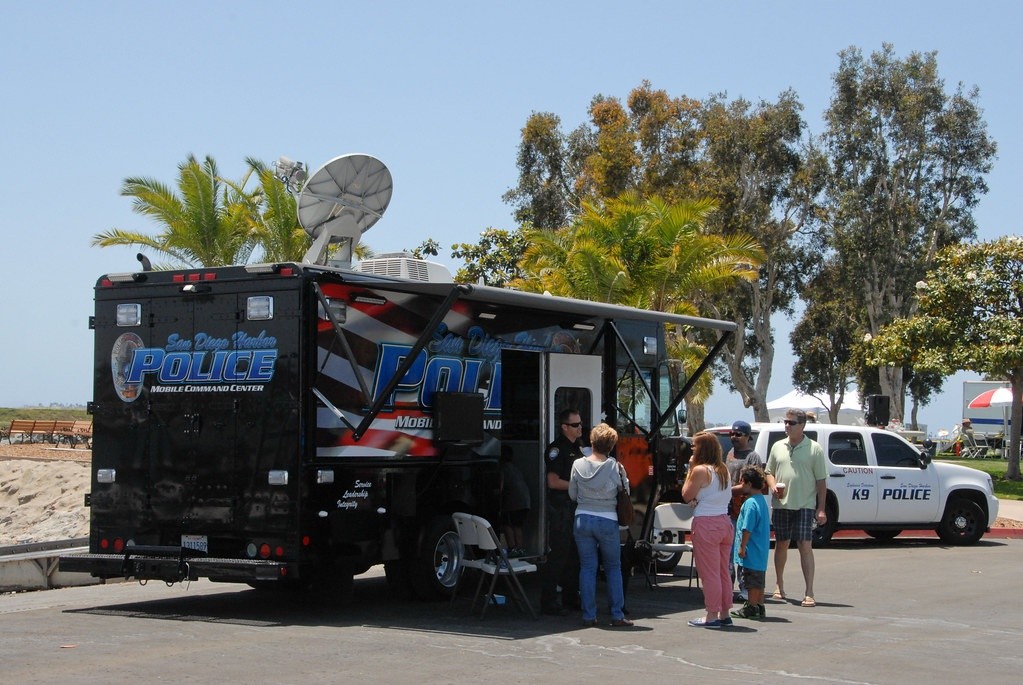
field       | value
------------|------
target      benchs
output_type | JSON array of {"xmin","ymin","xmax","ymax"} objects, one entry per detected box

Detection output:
[{"xmin": 0, "ymin": 419, "xmax": 93, "ymax": 449}]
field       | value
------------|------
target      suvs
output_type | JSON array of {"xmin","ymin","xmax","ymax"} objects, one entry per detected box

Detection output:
[{"xmin": 691, "ymin": 422, "xmax": 998, "ymax": 545}]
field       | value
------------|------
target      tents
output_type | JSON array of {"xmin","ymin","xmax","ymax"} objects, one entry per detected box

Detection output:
[{"xmin": 763, "ymin": 390, "xmax": 868, "ymax": 421}]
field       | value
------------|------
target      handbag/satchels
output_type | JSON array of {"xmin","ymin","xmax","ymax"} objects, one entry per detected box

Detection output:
[{"xmin": 616, "ymin": 473, "xmax": 634, "ymax": 527}]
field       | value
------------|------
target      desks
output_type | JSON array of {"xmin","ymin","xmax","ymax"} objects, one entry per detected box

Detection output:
[{"xmin": 987, "ymin": 437, "xmax": 1004, "ymax": 459}]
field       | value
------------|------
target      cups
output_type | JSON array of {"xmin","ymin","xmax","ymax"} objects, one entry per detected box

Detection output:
[{"xmin": 777, "ymin": 483, "xmax": 785, "ymax": 499}]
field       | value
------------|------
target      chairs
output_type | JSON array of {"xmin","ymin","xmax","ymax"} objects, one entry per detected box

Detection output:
[
  {"xmin": 597, "ymin": 503, "xmax": 699, "ymax": 591},
  {"xmin": 451, "ymin": 513, "xmax": 539, "ymax": 622},
  {"xmin": 961, "ymin": 433, "xmax": 1023, "ymax": 459}
]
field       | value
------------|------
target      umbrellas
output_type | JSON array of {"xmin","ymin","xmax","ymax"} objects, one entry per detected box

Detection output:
[{"xmin": 968, "ymin": 387, "xmax": 1023, "ymax": 435}]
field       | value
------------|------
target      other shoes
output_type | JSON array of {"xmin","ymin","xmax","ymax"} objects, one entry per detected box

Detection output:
[
  {"xmin": 772, "ymin": 590, "xmax": 785, "ymax": 599},
  {"xmin": 612, "ymin": 619, "xmax": 634, "ymax": 626},
  {"xmin": 562, "ymin": 599, "xmax": 581, "ymax": 612},
  {"xmin": 584, "ymin": 619, "xmax": 604, "ymax": 627},
  {"xmin": 543, "ymin": 603, "xmax": 566, "ymax": 616},
  {"xmin": 801, "ymin": 595, "xmax": 816, "ymax": 606}
]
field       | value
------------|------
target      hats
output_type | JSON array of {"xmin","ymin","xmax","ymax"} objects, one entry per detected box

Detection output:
[
  {"xmin": 961, "ymin": 419, "xmax": 971, "ymax": 423},
  {"xmin": 732, "ymin": 420, "xmax": 754, "ymax": 441},
  {"xmin": 806, "ymin": 411, "xmax": 816, "ymax": 418}
]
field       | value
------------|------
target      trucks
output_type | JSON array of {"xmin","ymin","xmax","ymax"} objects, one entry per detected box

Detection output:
[{"xmin": 84, "ymin": 253, "xmax": 738, "ymax": 604}]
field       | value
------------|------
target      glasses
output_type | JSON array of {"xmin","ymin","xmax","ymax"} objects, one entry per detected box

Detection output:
[
  {"xmin": 691, "ymin": 443, "xmax": 696, "ymax": 447},
  {"xmin": 565, "ymin": 421, "xmax": 582, "ymax": 428},
  {"xmin": 784, "ymin": 419, "xmax": 800, "ymax": 425},
  {"xmin": 729, "ymin": 431, "xmax": 746, "ymax": 437}
]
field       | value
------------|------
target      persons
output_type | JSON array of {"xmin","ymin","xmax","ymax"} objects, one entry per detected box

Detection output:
[
  {"xmin": 725, "ymin": 421, "xmax": 763, "ymax": 602},
  {"xmin": 543, "ymin": 410, "xmax": 582, "ymax": 616},
  {"xmin": 766, "ymin": 408, "xmax": 828, "ymax": 607},
  {"xmin": 731, "ymin": 465, "xmax": 770, "ymax": 620},
  {"xmin": 681, "ymin": 431, "xmax": 733, "ymax": 628},
  {"xmin": 569, "ymin": 423, "xmax": 633, "ymax": 626},
  {"xmin": 961, "ymin": 418, "xmax": 989, "ymax": 458},
  {"xmin": 499, "ymin": 446, "xmax": 531, "ymax": 560}
]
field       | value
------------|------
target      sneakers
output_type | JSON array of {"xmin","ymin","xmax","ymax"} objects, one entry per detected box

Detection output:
[
  {"xmin": 718, "ymin": 617, "xmax": 733, "ymax": 626},
  {"xmin": 688, "ymin": 617, "xmax": 720, "ymax": 628},
  {"xmin": 730, "ymin": 601, "xmax": 765, "ymax": 619}
]
